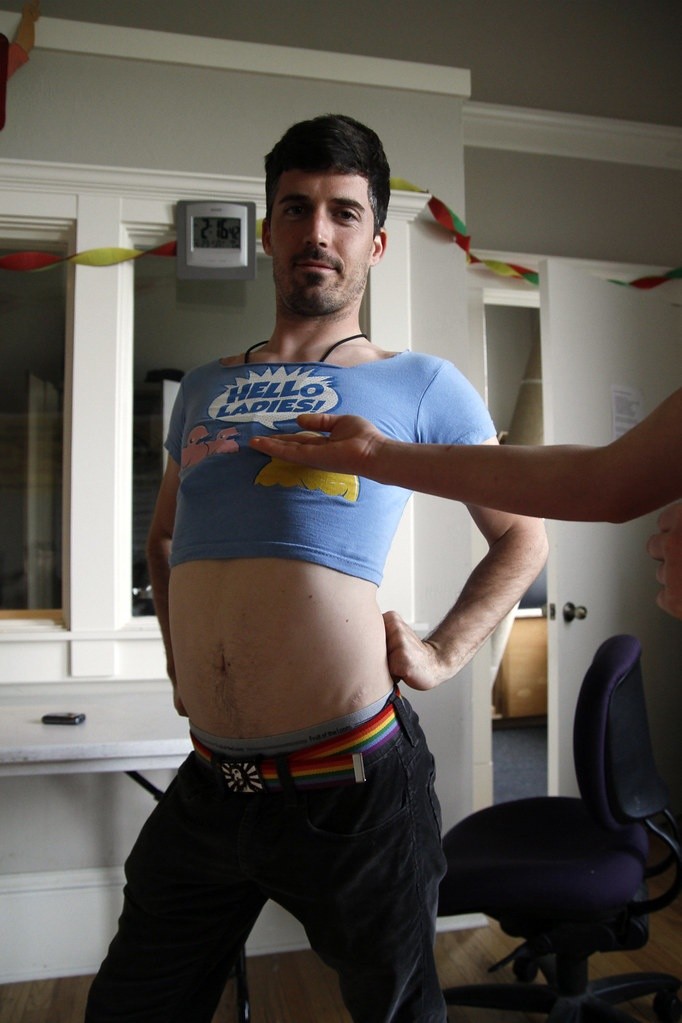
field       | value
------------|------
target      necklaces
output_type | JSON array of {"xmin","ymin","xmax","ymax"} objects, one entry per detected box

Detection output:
[{"xmin": 244, "ymin": 333, "xmax": 365, "ymax": 364}]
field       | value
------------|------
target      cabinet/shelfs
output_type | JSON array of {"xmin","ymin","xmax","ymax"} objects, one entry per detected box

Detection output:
[{"xmin": 133, "ymin": 379, "xmax": 181, "ymax": 481}]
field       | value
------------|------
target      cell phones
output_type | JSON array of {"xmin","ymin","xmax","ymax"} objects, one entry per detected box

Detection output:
[{"xmin": 41, "ymin": 712, "xmax": 85, "ymax": 724}]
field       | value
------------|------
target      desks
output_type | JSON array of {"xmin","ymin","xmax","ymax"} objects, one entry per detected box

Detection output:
[{"xmin": 0, "ymin": 684, "xmax": 249, "ymax": 1023}]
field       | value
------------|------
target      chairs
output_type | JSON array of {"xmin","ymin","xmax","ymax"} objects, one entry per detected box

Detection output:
[{"xmin": 438, "ymin": 635, "xmax": 682, "ymax": 1023}]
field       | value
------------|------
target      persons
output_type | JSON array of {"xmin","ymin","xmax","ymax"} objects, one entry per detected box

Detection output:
[
  {"xmin": 78, "ymin": 114, "xmax": 547, "ymax": 1023},
  {"xmin": 249, "ymin": 388, "xmax": 682, "ymax": 621}
]
